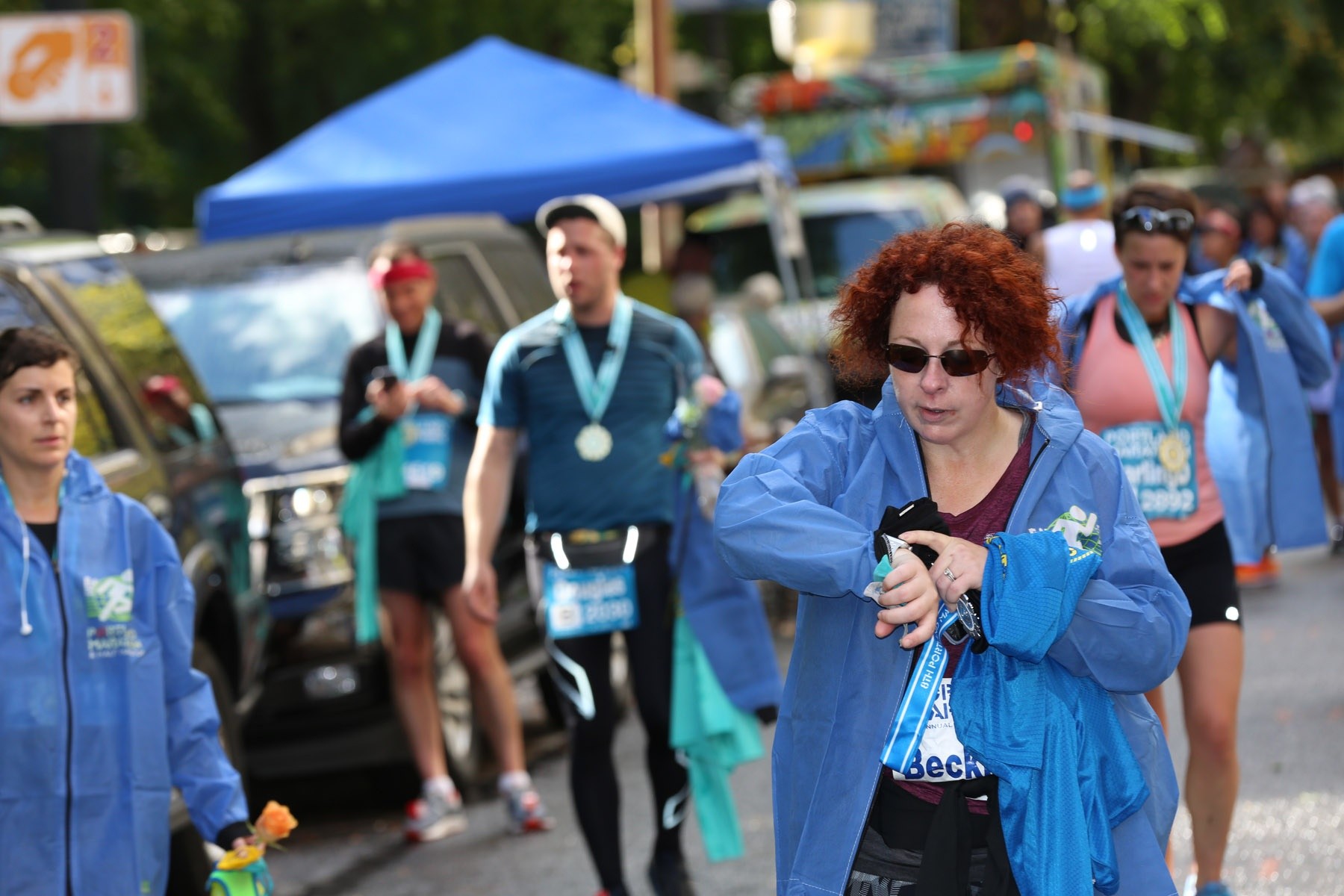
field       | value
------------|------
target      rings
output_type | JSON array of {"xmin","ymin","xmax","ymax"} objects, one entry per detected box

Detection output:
[{"xmin": 944, "ymin": 568, "xmax": 955, "ymax": 582}]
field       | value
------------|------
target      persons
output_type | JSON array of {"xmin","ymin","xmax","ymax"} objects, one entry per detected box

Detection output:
[
  {"xmin": 1054, "ymin": 182, "xmax": 1333, "ymax": 896},
  {"xmin": 998, "ymin": 169, "xmax": 1344, "ymax": 586},
  {"xmin": 336, "ymin": 242, "xmax": 553, "ymax": 842},
  {"xmin": 710, "ymin": 221, "xmax": 1193, "ymax": 896},
  {"xmin": 0, "ymin": 327, "xmax": 259, "ymax": 896},
  {"xmin": 462, "ymin": 194, "xmax": 725, "ymax": 895},
  {"xmin": 141, "ymin": 372, "xmax": 258, "ymax": 721}
]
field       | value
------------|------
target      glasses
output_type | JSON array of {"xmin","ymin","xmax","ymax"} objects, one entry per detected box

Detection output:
[
  {"xmin": 1122, "ymin": 205, "xmax": 1194, "ymax": 234},
  {"xmin": 884, "ymin": 341, "xmax": 998, "ymax": 378}
]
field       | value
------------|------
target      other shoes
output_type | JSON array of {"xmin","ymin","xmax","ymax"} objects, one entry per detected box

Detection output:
[
  {"xmin": 501, "ymin": 783, "xmax": 553, "ymax": 831},
  {"xmin": 1233, "ymin": 555, "xmax": 1279, "ymax": 588},
  {"xmin": 405, "ymin": 789, "xmax": 466, "ymax": 846},
  {"xmin": 1184, "ymin": 873, "xmax": 1232, "ymax": 896}
]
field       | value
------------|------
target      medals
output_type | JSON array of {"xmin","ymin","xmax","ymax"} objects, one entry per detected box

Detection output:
[
  {"xmin": 1159, "ymin": 428, "xmax": 1188, "ymax": 472},
  {"xmin": 405, "ymin": 423, "xmax": 418, "ymax": 445},
  {"xmin": 574, "ymin": 423, "xmax": 612, "ymax": 462}
]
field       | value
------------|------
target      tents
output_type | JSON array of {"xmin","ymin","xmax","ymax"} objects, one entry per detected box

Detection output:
[{"xmin": 191, "ymin": 35, "xmax": 831, "ymax": 411}]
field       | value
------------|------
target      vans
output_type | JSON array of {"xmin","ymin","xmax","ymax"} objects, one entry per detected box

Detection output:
[{"xmin": 0, "ymin": 36, "xmax": 1227, "ymax": 896}]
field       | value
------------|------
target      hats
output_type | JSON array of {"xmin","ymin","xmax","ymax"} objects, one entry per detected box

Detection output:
[{"xmin": 534, "ymin": 193, "xmax": 629, "ymax": 251}]
